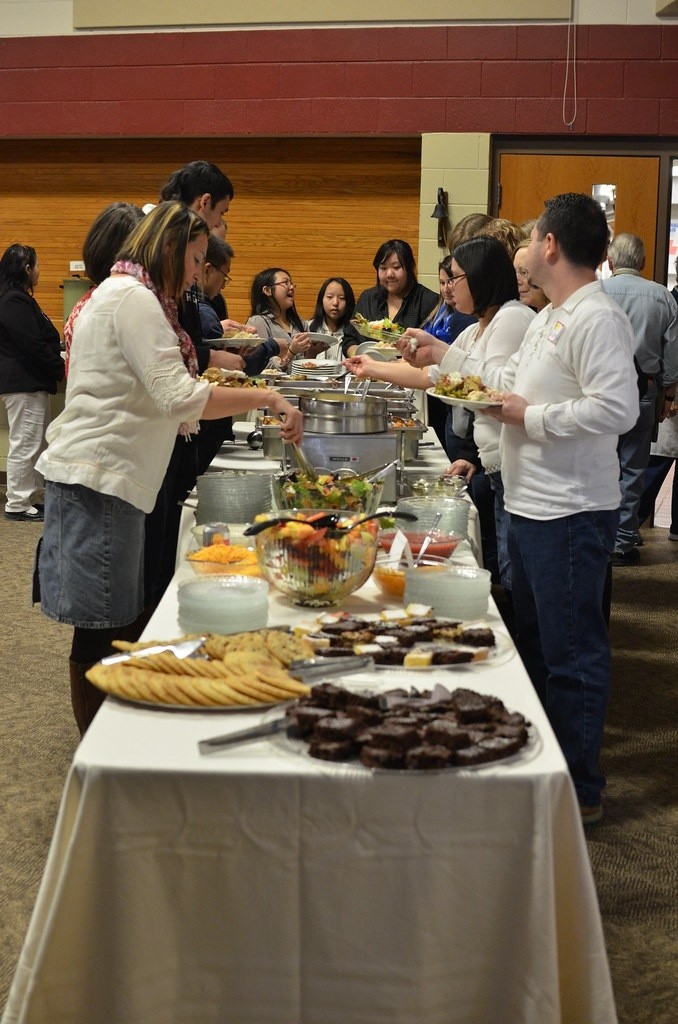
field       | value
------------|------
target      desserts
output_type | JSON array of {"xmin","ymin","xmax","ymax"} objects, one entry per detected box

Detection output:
[
  {"xmin": 292, "ymin": 603, "xmax": 496, "ymax": 667},
  {"xmin": 285, "ymin": 681, "xmax": 526, "ymax": 770}
]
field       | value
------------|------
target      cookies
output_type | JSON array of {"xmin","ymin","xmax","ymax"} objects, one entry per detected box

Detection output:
[{"xmin": 85, "ymin": 628, "xmax": 316, "ymax": 708}]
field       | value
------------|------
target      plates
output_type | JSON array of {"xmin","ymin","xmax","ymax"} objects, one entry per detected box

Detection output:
[
  {"xmin": 402, "ymin": 564, "xmax": 492, "ymax": 621},
  {"xmin": 258, "ymin": 372, "xmax": 287, "ymax": 380},
  {"xmin": 394, "ymin": 495, "xmax": 472, "ymax": 554},
  {"xmin": 350, "ymin": 320, "xmax": 411, "ymax": 344},
  {"xmin": 204, "ymin": 337, "xmax": 266, "ymax": 349},
  {"xmin": 366, "ymin": 616, "xmax": 515, "ymax": 674},
  {"xmin": 425, "ymin": 386, "xmax": 504, "ymax": 409},
  {"xmin": 355, "ymin": 341, "xmax": 397, "ymax": 362},
  {"xmin": 291, "ymin": 332, "xmax": 339, "ymax": 347},
  {"xmin": 195, "ymin": 473, "xmax": 279, "ymax": 525},
  {"xmin": 261, "ymin": 689, "xmax": 540, "ymax": 776},
  {"xmin": 176, "ymin": 573, "xmax": 270, "ymax": 639},
  {"xmin": 290, "ymin": 358, "xmax": 342, "ymax": 376}
]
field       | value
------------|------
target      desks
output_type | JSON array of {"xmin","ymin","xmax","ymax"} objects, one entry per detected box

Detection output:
[{"xmin": 1, "ymin": 419, "xmax": 621, "ymax": 1024}]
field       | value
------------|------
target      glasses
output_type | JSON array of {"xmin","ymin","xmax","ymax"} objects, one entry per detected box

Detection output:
[
  {"xmin": 516, "ymin": 266, "xmax": 529, "ymax": 279},
  {"xmin": 211, "ymin": 263, "xmax": 232, "ymax": 285},
  {"xmin": 447, "ymin": 274, "xmax": 467, "ymax": 287},
  {"xmin": 261, "ymin": 280, "xmax": 296, "ymax": 294}
]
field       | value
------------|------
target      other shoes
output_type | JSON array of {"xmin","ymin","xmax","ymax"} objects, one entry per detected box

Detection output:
[
  {"xmin": 611, "ymin": 547, "xmax": 641, "ymax": 567},
  {"xmin": 668, "ymin": 533, "xmax": 678, "ymax": 540},
  {"xmin": 6, "ymin": 504, "xmax": 45, "ymax": 522}
]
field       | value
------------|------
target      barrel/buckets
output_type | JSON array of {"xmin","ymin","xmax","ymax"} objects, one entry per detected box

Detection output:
[{"xmin": 298, "ymin": 393, "xmax": 388, "ymax": 434}]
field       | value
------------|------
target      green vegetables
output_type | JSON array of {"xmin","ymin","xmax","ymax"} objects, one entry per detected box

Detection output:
[
  {"xmin": 433, "ymin": 372, "xmax": 477, "ymax": 401},
  {"xmin": 351, "ymin": 312, "xmax": 404, "ymax": 341},
  {"xmin": 275, "ymin": 471, "xmax": 396, "ymax": 528}
]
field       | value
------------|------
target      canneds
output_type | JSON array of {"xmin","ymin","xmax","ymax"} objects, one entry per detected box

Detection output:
[{"xmin": 202, "ymin": 521, "xmax": 230, "ymax": 548}]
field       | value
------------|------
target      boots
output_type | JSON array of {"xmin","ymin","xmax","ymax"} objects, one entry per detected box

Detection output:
[{"xmin": 68, "ymin": 656, "xmax": 107, "ymax": 742}]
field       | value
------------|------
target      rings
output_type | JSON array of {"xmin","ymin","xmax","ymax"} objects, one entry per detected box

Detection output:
[{"xmin": 446, "ymin": 468, "xmax": 449, "ymax": 471}]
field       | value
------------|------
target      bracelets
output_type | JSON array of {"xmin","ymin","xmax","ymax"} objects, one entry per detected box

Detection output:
[
  {"xmin": 289, "ymin": 349, "xmax": 296, "ymax": 355},
  {"xmin": 664, "ymin": 395, "xmax": 675, "ymax": 402}
]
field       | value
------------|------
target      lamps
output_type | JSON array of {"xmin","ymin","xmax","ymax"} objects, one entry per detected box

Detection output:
[{"xmin": 431, "ymin": 187, "xmax": 451, "ymax": 248}]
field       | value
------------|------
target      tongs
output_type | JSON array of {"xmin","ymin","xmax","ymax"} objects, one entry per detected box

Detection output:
[
  {"xmin": 278, "ymin": 412, "xmax": 319, "ymax": 483},
  {"xmin": 287, "ymin": 651, "xmax": 375, "ymax": 684},
  {"xmin": 341, "ymin": 458, "xmax": 402, "ymax": 485}
]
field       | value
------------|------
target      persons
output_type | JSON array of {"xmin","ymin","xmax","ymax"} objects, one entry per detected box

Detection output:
[
  {"xmin": 341, "ymin": 239, "xmax": 440, "ymax": 359},
  {"xmin": 419, "ymin": 213, "xmax": 551, "ymax": 583},
  {"xmin": 143, "ymin": 160, "xmax": 289, "ymax": 611},
  {"xmin": 0, "ymin": 243, "xmax": 64, "ymax": 522},
  {"xmin": 340, "ymin": 235, "xmax": 537, "ymax": 642},
  {"xmin": 395, "ymin": 192, "xmax": 640, "ymax": 824},
  {"xmin": 245, "ymin": 268, "xmax": 329, "ymax": 375},
  {"xmin": 33, "ymin": 200, "xmax": 303, "ymax": 741},
  {"xmin": 307, "ymin": 277, "xmax": 355, "ymax": 362},
  {"xmin": 63, "ymin": 203, "xmax": 147, "ymax": 379},
  {"xmin": 601, "ymin": 233, "xmax": 678, "ymax": 565}
]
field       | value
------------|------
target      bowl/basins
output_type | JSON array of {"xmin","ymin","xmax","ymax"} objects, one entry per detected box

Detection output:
[
  {"xmin": 183, "ymin": 548, "xmax": 263, "ymax": 578},
  {"xmin": 254, "ymin": 509, "xmax": 380, "ymax": 609},
  {"xmin": 376, "ymin": 526, "xmax": 465, "ymax": 567},
  {"xmin": 405, "ymin": 472, "xmax": 469, "ymax": 499},
  {"xmin": 371, "ymin": 552, "xmax": 453, "ymax": 603}
]
